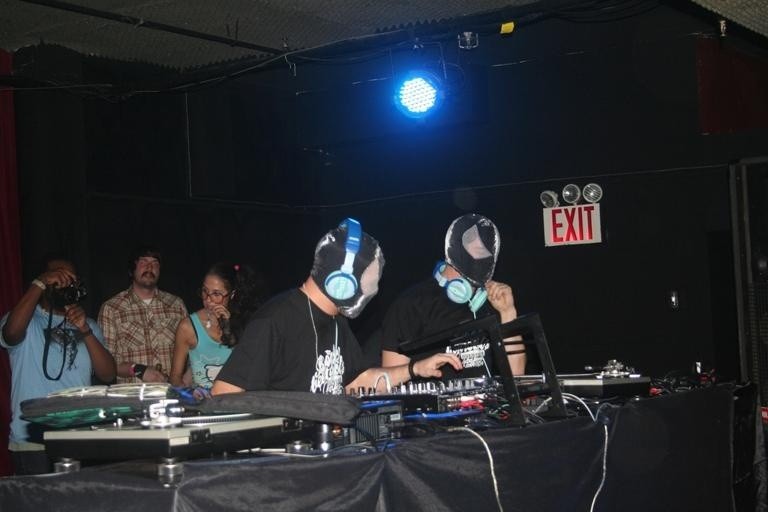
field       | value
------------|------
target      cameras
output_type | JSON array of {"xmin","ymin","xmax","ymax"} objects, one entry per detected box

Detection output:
[{"xmin": 44, "ymin": 279, "xmax": 87, "ymax": 307}]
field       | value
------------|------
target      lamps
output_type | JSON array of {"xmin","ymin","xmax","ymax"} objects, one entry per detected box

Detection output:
[
  {"xmin": 539, "ymin": 181, "xmax": 605, "ymax": 209},
  {"xmin": 387, "ymin": 40, "xmax": 452, "ymax": 122}
]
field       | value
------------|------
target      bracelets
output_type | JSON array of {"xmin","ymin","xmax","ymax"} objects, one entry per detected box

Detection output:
[
  {"xmin": 31, "ymin": 279, "xmax": 48, "ymax": 291},
  {"xmin": 409, "ymin": 358, "xmax": 420, "ymax": 380},
  {"xmin": 129, "ymin": 362, "xmax": 145, "ymax": 381},
  {"xmin": 82, "ymin": 329, "xmax": 93, "ymax": 337}
]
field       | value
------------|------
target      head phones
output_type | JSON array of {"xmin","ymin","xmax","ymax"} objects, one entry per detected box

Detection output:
[
  {"xmin": 324, "ymin": 217, "xmax": 363, "ymax": 300},
  {"xmin": 433, "ymin": 260, "xmax": 489, "ymax": 313}
]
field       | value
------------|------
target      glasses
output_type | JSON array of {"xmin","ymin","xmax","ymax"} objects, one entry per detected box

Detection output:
[{"xmin": 198, "ymin": 287, "xmax": 231, "ymax": 305}]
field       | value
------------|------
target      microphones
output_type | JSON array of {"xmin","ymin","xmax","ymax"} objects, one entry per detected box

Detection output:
[{"xmin": 465, "ymin": 296, "xmax": 476, "ymax": 319}]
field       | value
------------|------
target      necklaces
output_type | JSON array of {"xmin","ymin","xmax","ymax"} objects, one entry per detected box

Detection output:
[{"xmin": 206, "ymin": 312, "xmax": 212, "ymax": 329}]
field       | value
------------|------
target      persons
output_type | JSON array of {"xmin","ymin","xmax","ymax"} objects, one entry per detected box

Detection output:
[
  {"xmin": 381, "ymin": 215, "xmax": 528, "ymax": 378},
  {"xmin": 169, "ymin": 261, "xmax": 260, "ymax": 394},
  {"xmin": 210, "ymin": 218, "xmax": 463, "ymax": 404},
  {"xmin": 97, "ymin": 240, "xmax": 189, "ymax": 386},
  {"xmin": 0, "ymin": 250, "xmax": 117, "ymax": 474}
]
